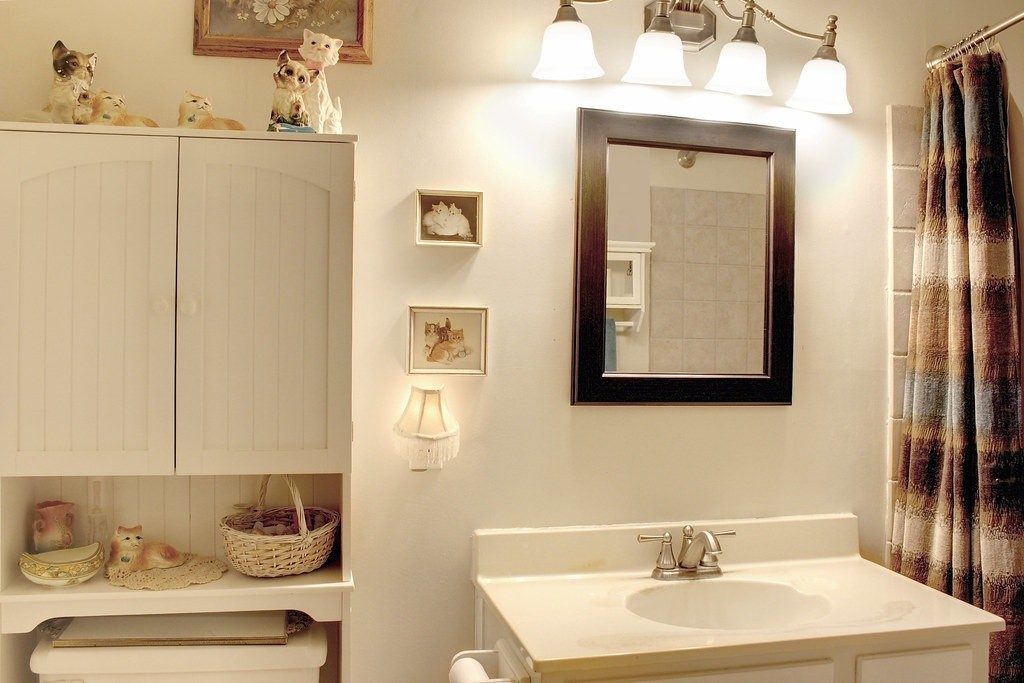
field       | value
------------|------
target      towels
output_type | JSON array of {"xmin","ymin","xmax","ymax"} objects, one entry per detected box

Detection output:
[{"xmin": 605, "ymin": 317, "xmax": 616, "ymax": 370}]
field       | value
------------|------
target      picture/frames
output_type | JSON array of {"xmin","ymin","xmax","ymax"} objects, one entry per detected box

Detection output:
[
  {"xmin": 568, "ymin": 107, "xmax": 799, "ymax": 406},
  {"xmin": 413, "ymin": 187, "xmax": 484, "ymax": 246},
  {"xmin": 193, "ymin": 0, "xmax": 376, "ymax": 67},
  {"xmin": 406, "ymin": 303, "xmax": 490, "ymax": 376}
]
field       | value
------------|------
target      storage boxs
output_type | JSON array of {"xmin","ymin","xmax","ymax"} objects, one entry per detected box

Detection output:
[{"xmin": 29, "ymin": 622, "xmax": 327, "ymax": 683}]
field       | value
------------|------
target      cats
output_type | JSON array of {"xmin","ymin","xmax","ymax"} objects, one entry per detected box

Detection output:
[
  {"xmin": 175, "ymin": 90, "xmax": 244, "ymax": 131},
  {"xmin": 266, "ymin": 28, "xmax": 344, "ymax": 134},
  {"xmin": 103, "ymin": 524, "xmax": 187, "ymax": 578},
  {"xmin": 16, "ymin": 40, "xmax": 161, "ymax": 127}
]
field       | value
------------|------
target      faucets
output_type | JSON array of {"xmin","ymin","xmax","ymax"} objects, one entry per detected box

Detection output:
[{"xmin": 678, "ymin": 525, "xmax": 722, "ymax": 579}]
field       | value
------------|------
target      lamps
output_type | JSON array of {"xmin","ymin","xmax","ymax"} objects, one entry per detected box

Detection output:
[
  {"xmin": 393, "ymin": 385, "xmax": 463, "ymax": 473},
  {"xmin": 530, "ymin": 0, "xmax": 607, "ymax": 82},
  {"xmin": 786, "ymin": 14, "xmax": 855, "ymax": 115},
  {"xmin": 702, "ymin": 0, "xmax": 773, "ymax": 98},
  {"xmin": 623, "ymin": 0, "xmax": 693, "ymax": 87}
]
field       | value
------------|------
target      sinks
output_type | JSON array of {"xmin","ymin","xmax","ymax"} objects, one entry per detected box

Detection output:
[{"xmin": 624, "ymin": 579, "xmax": 812, "ymax": 632}]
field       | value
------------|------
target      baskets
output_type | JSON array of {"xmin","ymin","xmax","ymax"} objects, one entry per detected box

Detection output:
[{"xmin": 219, "ymin": 474, "xmax": 341, "ymax": 577}]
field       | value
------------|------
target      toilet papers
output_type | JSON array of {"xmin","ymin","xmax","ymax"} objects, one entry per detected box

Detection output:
[{"xmin": 447, "ymin": 655, "xmax": 491, "ymax": 683}]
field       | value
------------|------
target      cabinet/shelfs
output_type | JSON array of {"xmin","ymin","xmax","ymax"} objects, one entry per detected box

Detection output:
[
  {"xmin": 605, "ymin": 236, "xmax": 657, "ymax": 331},
  {"xmin": 0, "ymin": 122, "xmax": 358, "ymax": 683},
  {"xmin": 478, "ymin": 601, "xmax": 989, "ymax": 681}
]
